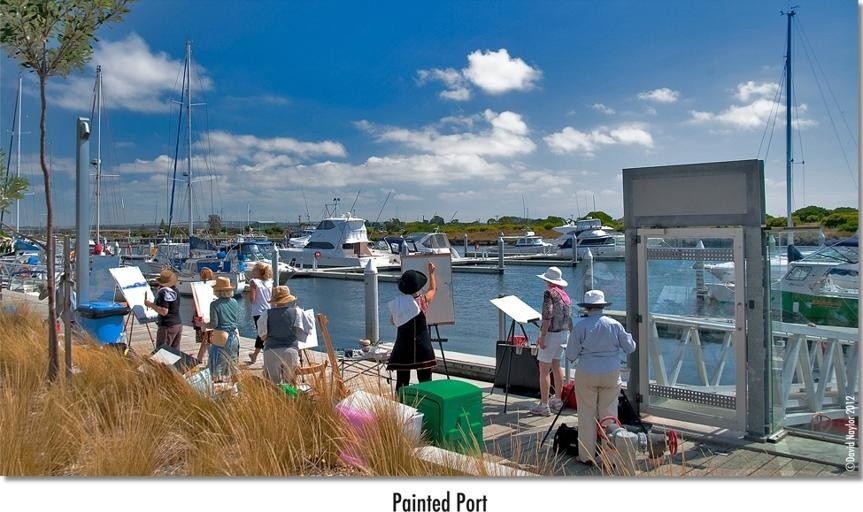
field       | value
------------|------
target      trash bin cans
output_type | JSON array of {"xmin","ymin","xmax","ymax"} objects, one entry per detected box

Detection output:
[{"xmin": 74, "ymin": 301, "xmax": 130, "ymax": 346}]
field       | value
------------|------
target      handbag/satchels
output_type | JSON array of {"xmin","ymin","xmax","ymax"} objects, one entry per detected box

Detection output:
[
  {"xmin": 553, "ymin": 423, "xmax": 577, "ymax": 458},
  {"xmin": 560, "ymin": 381, "xmax": 576, "ymax": 409}
]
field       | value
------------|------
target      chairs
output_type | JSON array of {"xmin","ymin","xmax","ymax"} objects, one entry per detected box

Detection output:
[{"xmin": 290, "ymin": 360, "xmax": 328, "ymax": 388}]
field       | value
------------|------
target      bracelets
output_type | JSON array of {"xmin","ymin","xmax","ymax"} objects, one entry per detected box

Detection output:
[{"xmin": 541, "ymin": 335, "xmax": 546, "ymax": 336}]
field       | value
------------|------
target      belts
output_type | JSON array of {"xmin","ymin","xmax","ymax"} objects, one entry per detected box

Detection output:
[{"xmin": 547, "ymin": 329, "xmax": 564, "ymax": 333}]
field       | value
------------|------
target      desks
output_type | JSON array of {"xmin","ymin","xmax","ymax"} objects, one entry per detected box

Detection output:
[{"xmin": 338, "ymin": 350, "xmax": 393, "ymax": 400}]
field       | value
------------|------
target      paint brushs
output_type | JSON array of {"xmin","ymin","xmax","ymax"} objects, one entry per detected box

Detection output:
[{"xmin": 146, "ymin": 307, "xmax": 149, "ymax": 311}]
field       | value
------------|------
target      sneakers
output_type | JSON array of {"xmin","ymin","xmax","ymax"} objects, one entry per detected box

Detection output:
[
  {"xmin": 547, "ymin": 399, "xmax": 562, "ymax": 412},
  {"xmin": 575, "ymin": 456, "xmax": 593, "ymax": 466},
  {"xmin": 248, "ymin": 352, "xmax": 256, "ymax": 364},
  {"xmin": 529, "ymin": 403, "xmax": 550, "ymax": 418}
]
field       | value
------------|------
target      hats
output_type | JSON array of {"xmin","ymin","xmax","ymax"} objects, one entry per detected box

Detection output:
[
  {"xmin": 211, "ymin": 277, "xmax": 236, "ymax": 291},
  {"xmin": 94, "ymin": 244, "xmax": 102, "ymax": 253},
  {"xmin": 156, "ymin": 270, "xmax": 177, "ymax": 287},
  {"xmin": 267, "ymin": 286, "xmax": 297, "ymax": 305},
  {"xmin": 575, "ymin": 290, "xmax": 612, "ymax": 309},
  {"xmin": 534, "ymin": 266, "xmax": 568, "ymax": 287},
  {"xmin": 397, "ymin": 270, "xmax": 427, "ymax": 294}
]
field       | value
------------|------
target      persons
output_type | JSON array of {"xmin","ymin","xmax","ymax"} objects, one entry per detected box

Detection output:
[
  {"xmin": 144, "ymin": 270, "xmax": 183, "ymax": 351},
  {"xmin": 257, "ymin": 285, "xmax": 300, "ymax": 385},
  {"xmin": 193, "ymin": 267, "xmax": 214, "ymax": 361},
  {"xmin": 386, "ymin": 261, "xmax": 438, "ymax": 392},
  {"xmin": 248, "ymin": 262, "xmax": 274, "ymax": 363},
  {"xmin": 529, "ymin": 266, "xmax": 573, "ymax": 417},
  {"xmin": 195, "ymin": 275, "xmax": 240, "ymax": 382},
  {"xmin": 565, "ymin": 289, "xmax": 637, "ymax": 467}
]
